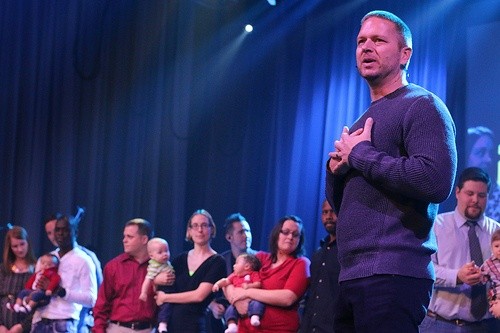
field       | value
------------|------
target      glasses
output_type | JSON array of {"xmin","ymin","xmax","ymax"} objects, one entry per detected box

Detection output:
[
  {"xmin": 279, "ymin": 229, "xmax": 301, "ymax": 238},
  {"xmin": 189, "ymin": 222, "xmax": 213, "ymax": 230}
]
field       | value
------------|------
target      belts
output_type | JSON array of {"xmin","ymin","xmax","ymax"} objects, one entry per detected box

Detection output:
[
  {"xmin": 427, "ymin": 309, "xmax": 491, "ymax": 327},
  {"xmin": 110, "ymin": 320, "xmax": 155, "ymax": 331},
  {"xmin": 37, "ymin": 317, "xmax": 76, "ymax": 326}
]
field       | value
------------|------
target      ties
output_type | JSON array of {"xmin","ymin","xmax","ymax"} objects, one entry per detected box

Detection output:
[{"xmin": 465, "ymin": 220, "xmax": 488, "ymax": 320}]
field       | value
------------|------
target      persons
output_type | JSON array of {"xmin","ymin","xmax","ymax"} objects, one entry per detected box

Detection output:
[
  {"xmin": 207, "ymin": 212, "xmax": 258, "ymax": 319},
  {"xmin": 488, "ymin": 304, "xmax": 500, "ymax": 320},
  {"xmin": 301, "ymin": 197, "xmax": 353, "ymax": 333},
  {"xmin": 224, "ymin": 215, "xmax": 312, "ymax": 333},
  {"xmin": 31, "ymin": 217, "xmax": 103, "ymax": 333},
  {"xmin": 92, "ymin": 219, "xmax": 157, "ymax": 333},
  {"xmin": 0, "ymin": 225, "xmax": 37, "ymax": 333},
  {"xmin": 14, "ymin": 253, "xmax": 59, "ymax": 314},
  {"xmin": 210, "ymin": 254, "xmax": 266, "ymax": 333},
  {"xmin": 34, "ymin": 219, "xmax": 104, "ymax": 297},
  {"xmin": 419, "ymin": 167, "xmax": 499, "ymax": 333},
  {"xmin": 325, "ymin": 10, "xmax": 457, "ymax": 333},
  {"xmin": 153, "ymin": 209, "xmax": 227, "ymax": 333},
  {"xmin": 141, "ymin": 237, "xmax": 175, "ymax": 333},
  {"xmin": 471, "ymin": 229, "xmax": 499, "ymax": 320}
]
